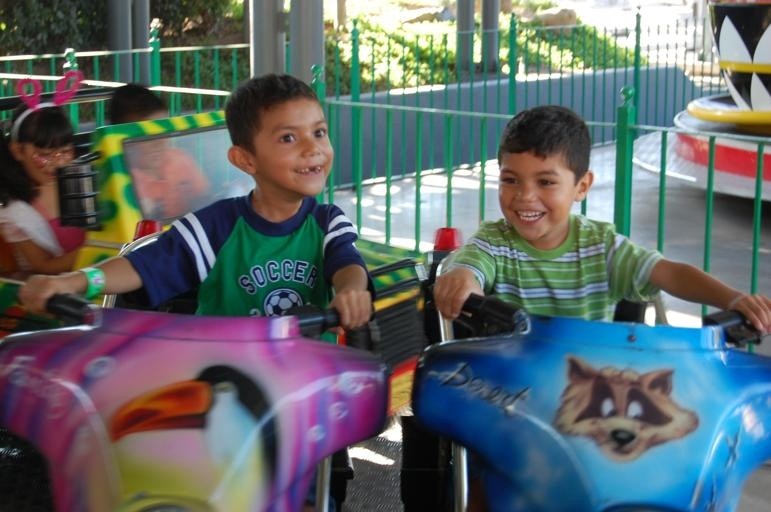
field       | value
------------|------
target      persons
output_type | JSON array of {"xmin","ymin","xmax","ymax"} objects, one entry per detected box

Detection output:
[
  {"xmin": 0, "ymin": 71, "xmax": 87, "ymax": 280},
  {"xmin": 110, "ymin": 82, "xmax": 211, "ymax": 223},
  {"xmin": 19, "ymin": 73, "xmax": 376, "ymax": 330},
  {"xmin": 433, "ymin": 104, "xmax": 771, "ymax": 336}
]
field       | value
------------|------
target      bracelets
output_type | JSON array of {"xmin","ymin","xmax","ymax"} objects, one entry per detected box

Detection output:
[
  {"xmin": 728, "ymin": 292, "xmax": 747, "ymax": 311},
  {"xmin": 80, "ymin": 266, "xmax": 106, "ymax": 300}
]
